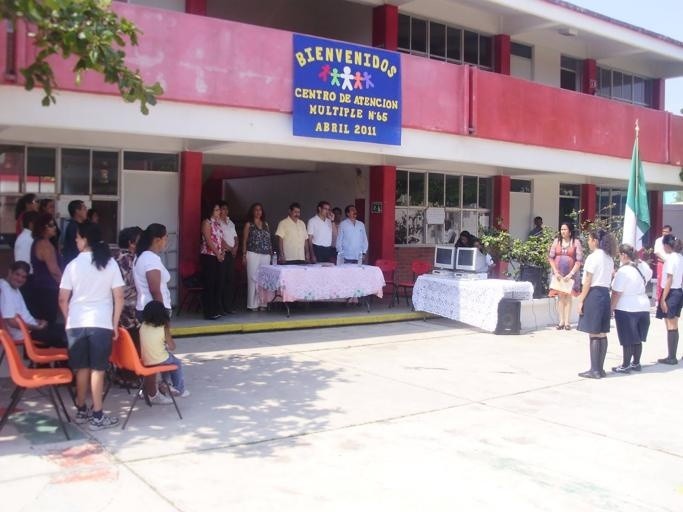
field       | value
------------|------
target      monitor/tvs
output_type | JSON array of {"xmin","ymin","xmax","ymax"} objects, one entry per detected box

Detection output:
[
  {"xmin": 454, "ymin": 247, "xmax": 488, "ymax": 280},
  {"xmin": 432, "ymin": 245, "xmax": 456, "ymax": 276}
]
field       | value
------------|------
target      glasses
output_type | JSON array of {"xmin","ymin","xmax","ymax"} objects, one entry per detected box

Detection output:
[{"xmin": 47, "ymin": 222, "xmax": 56, "ymax": 227}]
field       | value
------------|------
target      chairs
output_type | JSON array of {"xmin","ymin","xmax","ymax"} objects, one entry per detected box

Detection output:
[
  {"xmin": 9, "ymin": 313, "xmax": 74, "ymax": 401},
  {"xmin": 0, "ymin": 329, "xmax": 73, "ymax": 440},
  {"xmin": 388, "ymin": 261, "xmax": 429, "ymax": 311},
  {"xmin": 102, "ymin": 327, "xmax": 183, "ymax": 429},
  {"xmin": 0, "ymin": 310, "xmax": 44, "ymax": 366},
  {"xmin": 371, "ymin": 259, "xmax": 399, "ymax": 306}
]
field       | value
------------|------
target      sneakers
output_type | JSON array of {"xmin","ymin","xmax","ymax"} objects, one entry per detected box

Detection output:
[
  {"xmin": 556, "ymin": 324, "xmax": 677, "ymax": 378},
  {"xmin": 75, "ymin": 382, "xmax": 190, "ymax": 431}
]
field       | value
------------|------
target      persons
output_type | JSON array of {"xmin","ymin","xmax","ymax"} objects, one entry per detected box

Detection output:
[
  {"xmin": 548, "ymin": 221, "xmax": 583, "ymax": 330},
  {"xmin": 275, "ymin": 202, "xmax": 311, "ymax": 264},
  {"xmin": 335, "ymin": 204, "xmax": 369, "ymax": 307},
  {"xmin": 200, "ymin": 200, "xmax": 273, "ymax": 320},
  {"xmin": 1, "ymin": 192, "xmax": 192, "ymax": 429},
  {"xmin": 455, "ymin": 215, "xmax": 545, "ymax": 267},
  {"xmin": 307, "ymin": 200, "xmax": 337, "ymax": 263},
  {"xmin": 329, "ymin": 207, "xmax": 343, "ymax": 264},
  {"xmin": 576, "ymin": 224, "xmax": 683, "ymax": 378},
  {"xmin": 445, "ymin": 218, "xmax": 456, "ymax": 243}
]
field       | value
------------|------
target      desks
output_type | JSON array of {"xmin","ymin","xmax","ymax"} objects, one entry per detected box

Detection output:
[
  {"xmin": 412, "ymin": 273, "xmax": 534, "ymax": 332},
  {"xmin": 250, "ymin": 263, "xmax": 386, "ymax": 318}
]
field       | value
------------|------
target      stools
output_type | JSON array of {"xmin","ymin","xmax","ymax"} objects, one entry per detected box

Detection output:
[{"xmin": 176, "ymin": 288, "xmax": 205, "ymax": 316}]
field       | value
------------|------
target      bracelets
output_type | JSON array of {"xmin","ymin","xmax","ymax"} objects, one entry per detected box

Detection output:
[
  {"xmin": 570, "ymin": 270, "xmax": 575, "ymax": 276},
  {"xmin": 331, "ymin": 219, "xmax": 335, "ymax": 222},
  {"xmin": 553, "ymin": 269, "xmax": 559, "ymax": 275}
]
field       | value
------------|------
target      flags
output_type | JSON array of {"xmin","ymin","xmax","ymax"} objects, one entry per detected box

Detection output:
[{"xmin": 622, "ymin": 137, "xmax": 651, "ymax": 250}]
